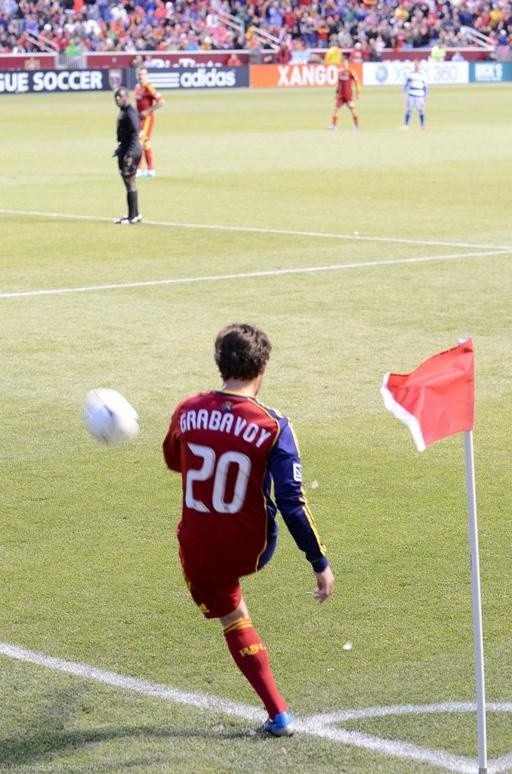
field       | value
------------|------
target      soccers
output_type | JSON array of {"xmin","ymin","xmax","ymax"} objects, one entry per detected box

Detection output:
[{"xmin": 85, "ymin": 388, "xmax": 140, "ymax": 447}]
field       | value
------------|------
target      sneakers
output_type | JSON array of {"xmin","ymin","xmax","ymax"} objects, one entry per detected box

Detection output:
[
  {"xmin": 116, "ymin": 215, "xmax": 142, "ymax": 224},
  {"xmin": 264, "ymin": 710, "xmax": 295, "ymax": 738}
]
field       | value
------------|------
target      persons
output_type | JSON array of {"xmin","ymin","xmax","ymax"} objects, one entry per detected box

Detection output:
[
  {"xmin": 400, "ymin": 63, "xmax": 430, "ymax": 131},
  {"xmin": 130, "ymin": 64, "xmax": 165, "ymax": 179},
  {"xmin": 0, "ymin": 2, "xmax": 512, "ymax": 67},
  {"xmin": 328, "ymin": 57, "xmax": 362, "ymax": 133},
  {"xmin": 111, "ymin": 89, "xmax": 146, "ymax": 227},
  {"xmin": 157, "ymin": 323, "xmax": 338, "ymax": 742}
]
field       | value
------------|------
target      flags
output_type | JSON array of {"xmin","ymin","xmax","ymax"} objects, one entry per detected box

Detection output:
[{"xmin": 378, "ymin": 337, "xmax": 477, "ymax": 455}]
